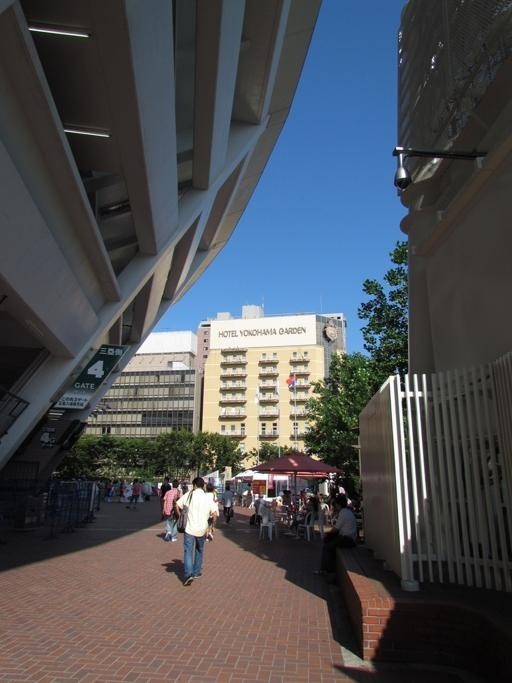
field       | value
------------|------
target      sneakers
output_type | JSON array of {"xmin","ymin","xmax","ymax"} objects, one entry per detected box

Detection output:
[
  {"xmin": 183, "ymin": 577, "xmax": 193, "ymax": 586},
  {"xmin": 312, "ymin": 568, "xmax": 328, "ymax": 576},
  {"xmin": 192, "ymin": 573, "xmax": 202, "ymax": 579},
  {"xmin": 164, "ymin": 532, "xmax": 177, "ymax": 542}
]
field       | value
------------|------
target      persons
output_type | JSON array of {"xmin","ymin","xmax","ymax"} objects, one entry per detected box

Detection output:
[
  {"xmin": 105, "ymin": 478, "xmax": 153, "ymax": 509},
  {"xmin": 174, "ymin": 477, "xmax": 220, "ymax": 585},
  {"xmin": 53, "ymin": 475, "xmax": 107, "ymax": 514},
  {"xmin": 313, "ymin": 494, "xmax": 359, "ymax": 576},
  {"xmin": 255, "ymin": 495, "xmax": 264, "ymax": 522},
  {"xmin": 224, "ymin": 485, "xmax": 233, "ymax": 515},
  {"xmin": 160, "ymin": 476, "xmax": 188, "ymax": 540},
  {"xmin": 206, "ymin": 482, "xmax": 219, "ymax": 540},
  {"xmin": 282, "ymin": 489, "xmax": 361, "ymax": 531}
]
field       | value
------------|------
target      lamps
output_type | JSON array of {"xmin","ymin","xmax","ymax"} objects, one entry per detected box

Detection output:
[
  {"xmin": 393, "ymin": 146, "xmax": 488, "ymax": 192},
  {"xmin": 28, "ymin": 24, "xmax": 91, "ymax": 39},
  {"xmin": 64, "ymin": 123, "xmax": 112, "ymax": 138}
]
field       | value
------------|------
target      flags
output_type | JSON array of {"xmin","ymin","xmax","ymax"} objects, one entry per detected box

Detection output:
[{"xmin": 286, "ymin": 374, "xmax": 296, "ymax": 385}]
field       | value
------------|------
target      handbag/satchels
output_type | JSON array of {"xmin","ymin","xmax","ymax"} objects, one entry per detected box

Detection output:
[{"xmin": 229, "ymin": 508, "xmax": 234, "ymax": 517}]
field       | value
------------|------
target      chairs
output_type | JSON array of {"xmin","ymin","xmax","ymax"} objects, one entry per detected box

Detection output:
[{"xmin": 258, "ymin": 508, "xmax": 326, "ymax": 544}]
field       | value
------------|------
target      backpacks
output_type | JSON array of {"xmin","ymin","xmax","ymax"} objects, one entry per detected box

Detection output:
[
  {"xmin": 173, "ymin": 490, "xmax": 180, "ymax": 516},
  {"xmin": 176, "ymin": 513, "xmax": 185, "ymax": 532}
]
